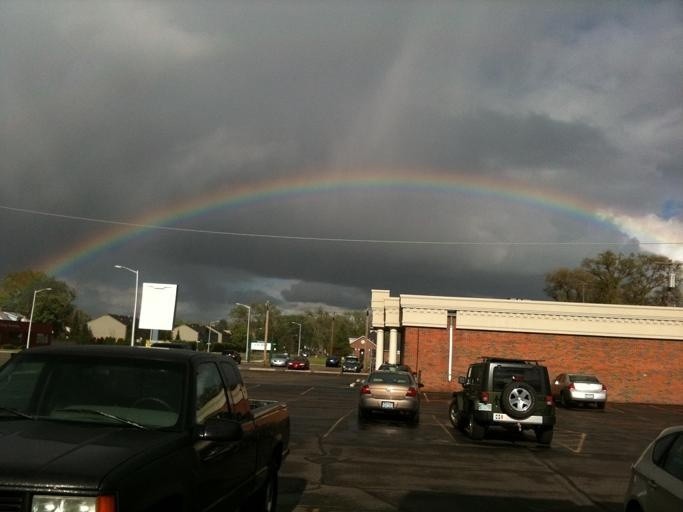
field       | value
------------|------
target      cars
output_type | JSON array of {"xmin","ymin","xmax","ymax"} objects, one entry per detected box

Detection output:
[
  {"xmin": 342, "ymin": 356, "xmax": 362, "ymax": 372},
  {"xmin": 624, "ymin": 425, "xmax": 682, "ymax": 512},
  {"xmin": 288, "ymin": 355, "xmax": 309, "ymax": 370},
  {"xmin": 550, "ymin": 373, "xmax": 606, "ymax": 409},
  {"xmin": 326, "ymin": 356, "xmax": 341, "ymax": 367},
  {"xmin": 270, "ymin": 354, "xmax": 289, "ymax": 367},
  {"xmin": 222, "ymin": 350, "xmax": 241, "ymax": 365},
  {"xmin": 378, "ymin": 363, "xmax": 417, "ymax": 379},
  {"xmin": 358, "ymin": 370, "xmax": 420, "ymax": 427}
]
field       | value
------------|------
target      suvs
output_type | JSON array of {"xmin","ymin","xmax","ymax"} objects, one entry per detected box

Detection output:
[{"xmin": 448, "ymin": 354, "xmax": 556, "ymax": 447}]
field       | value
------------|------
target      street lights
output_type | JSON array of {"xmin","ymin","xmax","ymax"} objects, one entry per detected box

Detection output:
[
  {"xmin": 114, "ymin": 265, "xmax": 138, "ymax": 347},
  {"xmin": 291, "ymin": 322, "xmax": 302, "ymax": 356},
  {"xmin": 26, "ymin": 287, "xmax": 52, "ymax": 349},
  {"xmin": 235, "ymin": 303, "xmax": 250, "ymax": 363}
]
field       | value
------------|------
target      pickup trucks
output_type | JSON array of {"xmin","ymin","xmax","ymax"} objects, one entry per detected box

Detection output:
[{"xmin": 0, "ymin": 342, "xmax": 290, "ymax": 512}]
